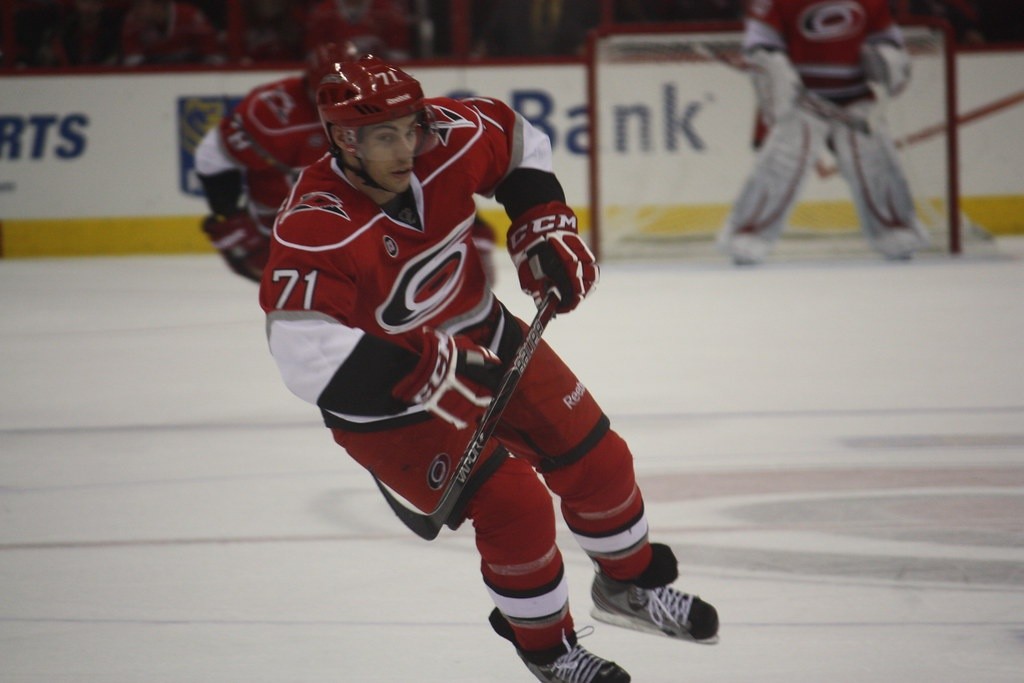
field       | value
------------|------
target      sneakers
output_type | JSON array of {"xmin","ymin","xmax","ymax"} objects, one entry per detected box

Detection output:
[
  {"xmin": 489, "ymin": 607, "xmax": 631, "ymax": 683},
  {"xmin": 590, "ymin": 543, "xmax": 720, "ymax": 645}
]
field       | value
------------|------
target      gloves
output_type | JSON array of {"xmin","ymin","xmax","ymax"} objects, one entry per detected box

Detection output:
[
  {"xmin": 391, "ymin": 326, "xmax": 505, "ymax": 431},
  {"xmin": 203, "ymin": 212, "xmax": 273, "ymax": 283},
  {"xmin": 506, "ymin": 200, "xmax": 600, "ymax": 318}
]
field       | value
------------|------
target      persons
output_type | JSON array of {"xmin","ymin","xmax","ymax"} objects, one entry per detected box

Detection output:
[
  {"xmin": 257, "ymin": 54, "xmax": 720, "ymax": 682},
  {"xmin": 192, "ymin": 38, "xmax": 395, "ymax": 283},
  {"xmin": 125, "ymin": 2, "xmax": 409, "ymax": 71},
  {"xmin": 720, "ymin": 0, "xmax": 932, "ymax": 268}
]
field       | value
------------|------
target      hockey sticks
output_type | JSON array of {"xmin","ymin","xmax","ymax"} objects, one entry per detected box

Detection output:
[{"xmin": 366, "ymin": 272, "xmax": 564, "ymax": 542}]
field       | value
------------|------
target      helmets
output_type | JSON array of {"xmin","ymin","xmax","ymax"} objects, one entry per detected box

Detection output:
[
  {"xmin": 307, "ymin": 42, "xmax": 354, "ymax": 93},
  {"xmin": 315, "ymin": 54, "xmax": 439, "ymax": 163}
]
